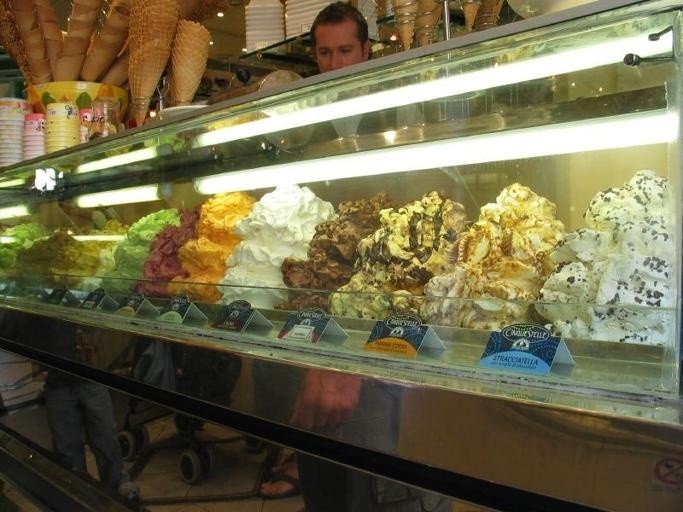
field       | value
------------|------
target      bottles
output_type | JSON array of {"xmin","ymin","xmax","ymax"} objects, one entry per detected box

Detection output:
[{"xmin": 85, "ymin": 101, "xmax": 124, "ymax": 144}]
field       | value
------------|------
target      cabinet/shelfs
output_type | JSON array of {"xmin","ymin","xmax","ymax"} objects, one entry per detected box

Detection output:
[{"xmin": 0, "ymin": 1, "xmax": 683, "ymax": 509}]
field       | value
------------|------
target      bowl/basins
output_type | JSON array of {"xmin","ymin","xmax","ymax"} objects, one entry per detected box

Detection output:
[
  {"xmin": 45, "ymin": 102, "xmax": 80, "ymax": 156},
  {"xmin": 22, "ymin": 113, "xmax": 45, "ymax": 161},
  {"xmin": 0, "ymin": 96, "xmax": 33, "ymax": 168},
  {"xmin": 26, "ymin": 81, "xmax": 130, "ymax": 121}
]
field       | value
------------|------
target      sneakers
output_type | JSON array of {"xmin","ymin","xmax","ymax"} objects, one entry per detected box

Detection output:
[{"xmin": 115, "ymin": 481, "xmax": 140, "ymax": 506}]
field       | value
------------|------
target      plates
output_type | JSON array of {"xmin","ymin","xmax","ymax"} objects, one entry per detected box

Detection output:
[{"xmin": 157, "ymin": 104, "xmax": 209, "ymax": 120}]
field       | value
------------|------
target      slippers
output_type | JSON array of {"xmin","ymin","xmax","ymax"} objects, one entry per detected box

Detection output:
[{"xmin": 258, "ymin": 474, "xmax": 301, "ymax": 498}]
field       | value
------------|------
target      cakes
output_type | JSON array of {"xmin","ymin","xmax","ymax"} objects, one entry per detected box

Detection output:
[
  {"xmin": 420, "ymin": 179, "xmax": 566, "ymax": 335},
  {"xmin": 532, "ymin": 164, "xmax": 676, "ymax": 351},
  {"xmin": 47, "ymin": 218, "xmax": 130, "ymax": 286},
  {"xmin": 100, "ymin": 207, "xmax": 181, "ymax": 295},
  {"xmin": 164, "ymin": 189, "xmax": 258, "ymax": 305},
  {"xmin": 329, "ymin": 190, "xmax": 468, "ymax": 329},
  {"xmin": 132, "ymin": 200, "xmax": 205, "ymax": 300},
  {"xmin": 6, "ymin": 223, "xmax": 94, "ymax": 289},
  {"xmin": 273, "ymin": 191, "xmax": 407, "ymax": 317},
  {"xmin": 0, "ymin": 219, "xmax": 47, "ymax": 274},
  {"xmin": 214, "ymin": 182, "xmax": 339, "ymax": 315}
]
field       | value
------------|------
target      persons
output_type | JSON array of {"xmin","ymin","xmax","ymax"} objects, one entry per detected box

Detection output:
[
  {"xmin": 253, "ymin": 360, "xmax": 305, "ymax": 498},
  {"xmin": 288, "ymin": 367, "xmax": 452, "ymax": 512},
  {"xmin": 44, "ymin": 365, "xmax": 141, "ymax": 508},
  {"xmin": 264, "ymin": 3, "xmax": 425, "ymax": 203}
]
